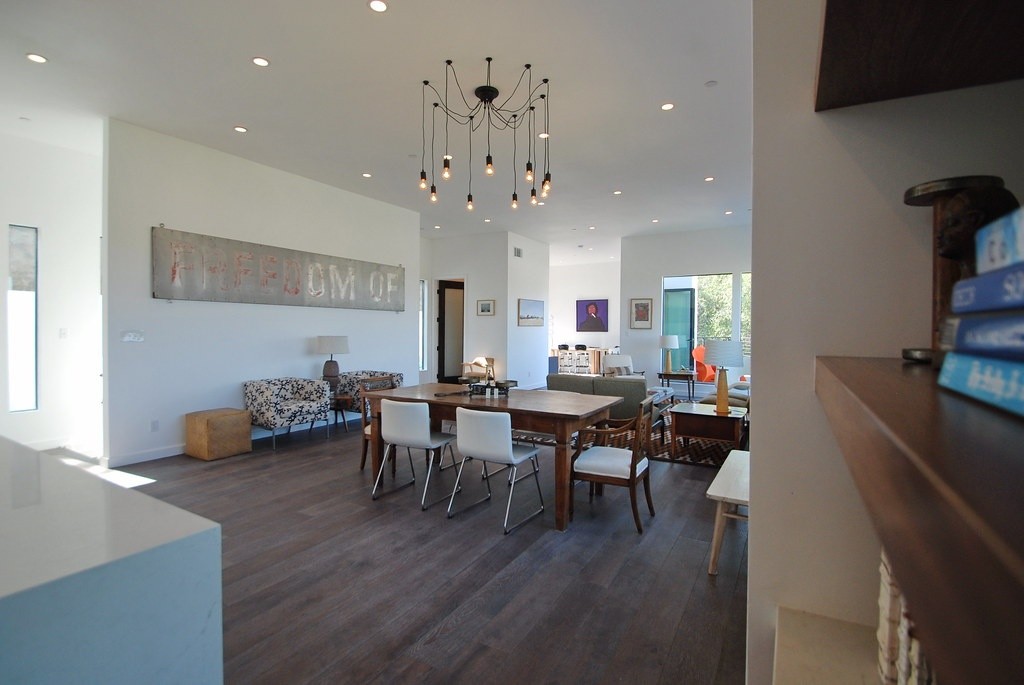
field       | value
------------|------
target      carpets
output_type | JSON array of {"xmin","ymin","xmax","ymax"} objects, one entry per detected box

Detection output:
[{"xmin": 512, "ymin": 396, "xmax": 748, "ymax": 464}]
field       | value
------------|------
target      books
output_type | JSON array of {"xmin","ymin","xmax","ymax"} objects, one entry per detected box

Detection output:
[
  {"xmin": 936, "ymin": 204, "xmax": 1024, "ymax": 416},
  {"xmin": 876, "ymin": 543, "xmax": 937, "ymax": 685}
]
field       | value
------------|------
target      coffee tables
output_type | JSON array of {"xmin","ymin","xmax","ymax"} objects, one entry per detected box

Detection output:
[{"xmin": 647, "ymin": 386, "xmax": 675, "ymax": 407}]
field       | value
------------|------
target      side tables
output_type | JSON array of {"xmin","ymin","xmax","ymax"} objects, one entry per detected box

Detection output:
[{"xmin": 670, "ymin": 402, "xmax": 748, "ymax": 461}]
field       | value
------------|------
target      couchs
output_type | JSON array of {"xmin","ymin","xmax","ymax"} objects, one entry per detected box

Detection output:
[
  {"xmin": 701, "ymin": 380, "xmax": 751, "ymax": 408},
  {"xmin": 546, "ymin": 373, "xmax": 667, "ymax": 429}
]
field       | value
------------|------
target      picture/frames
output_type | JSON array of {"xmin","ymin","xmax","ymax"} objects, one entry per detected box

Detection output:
[
  {"xmin": 630, "ymin": 297, "xmax": 653, "ymax": 328},
  {"xmin": 576, "ymin": 300, "xmax": 609, "ymax": 332},
  {"xmin": 518, "ymin": 299, "xmax": 546, "ymax": 326},
  {"xmin": 477, "ymin": 300, "xmax": 495, "ymax": 316}
]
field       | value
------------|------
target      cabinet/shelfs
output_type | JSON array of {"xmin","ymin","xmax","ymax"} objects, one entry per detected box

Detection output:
[{"xmin": 816, "ymin": 1, "xmax": 1024, "ymax": 685}]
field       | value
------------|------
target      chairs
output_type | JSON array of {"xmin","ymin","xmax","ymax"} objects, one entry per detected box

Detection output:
[
  {"xmin": 243, "ymin": 378, "xmax": 332, "ymax": 451},
  {"xmin": 570, "ymin": 396, "xmax": 657, "ymax": 534},
  {"xmin": 372, "ymin": 400, "xmax": 463, "ymax": 511},
  {"xmin": 601, "ymin": 355, "xmax": 645, "ymax": 377},
  {"xmin": 334, "ymin": 369, "xmax": 404, "ymax": 424},
  {"xmin": 448, "ymin": 405, "xmax": 546, "ymax": 535},
  {"xmin": 508, "ymin": 430, "xmax": 580, "ymax": 485},
  {"xmin": 361, "ymin": 374, "xmax": 399, "ymax": 478}
]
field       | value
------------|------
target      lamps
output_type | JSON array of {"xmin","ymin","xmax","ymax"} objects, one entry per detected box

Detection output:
[
  {"xmin": 659, "ymin": 334, "xmax": 679, "ymax": 371},
  {"xmin": 315, "ymin": 335, "xmax": 350, "ymax": 393},
  {"xmin": 416, "ymin": 58, "xmax": 551, "ymax": 209}
]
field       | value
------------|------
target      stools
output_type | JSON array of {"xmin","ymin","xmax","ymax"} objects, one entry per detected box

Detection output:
[{"xmin": 184, "ymin": 407, "xmax": 254, "ymax": 462}]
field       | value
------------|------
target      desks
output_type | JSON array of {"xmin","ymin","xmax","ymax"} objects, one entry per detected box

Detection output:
[
  {"xmin": 658, "ymin": 371, "xmax": 699, "ymax": 399},
  {"xmin": 364, "ymin": 382, "xmax": 623, "ymax": 534},
  {"xmin": 707, "ymin": 448, "xmax": 749, "ymax": 574},
  {"xmin": 314, "ymin": 396, "xmax": 352, "ymax": 434}
]
